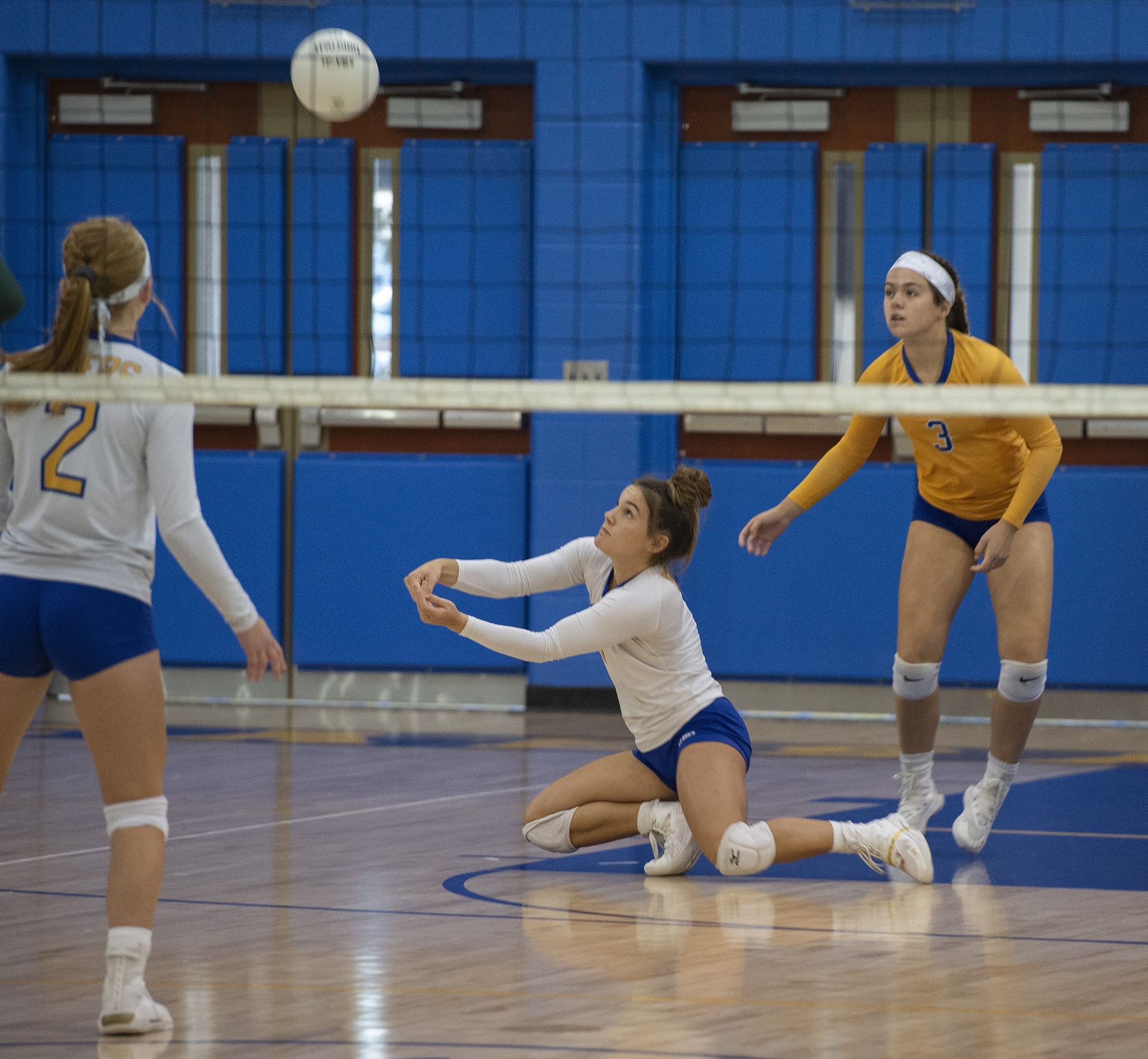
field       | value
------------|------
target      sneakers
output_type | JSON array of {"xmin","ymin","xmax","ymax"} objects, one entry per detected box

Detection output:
[
  {"xmin": 638, "ymin": 797, "xmax": 702, "ymax": 875},
  {"xmin": 95, "ymin": 934, "xmax": 174, "ymax": 1035},
  {"xmin": 891, "ymin": 761, "xmax": 945, "ymax": 836},
  {"xmin": 840, "ymin": 813, "xmax": 934, "ymax": 884},
  {"xmin": 951, "ymin": 771, "xmax": 1011, "ymax": 854}
]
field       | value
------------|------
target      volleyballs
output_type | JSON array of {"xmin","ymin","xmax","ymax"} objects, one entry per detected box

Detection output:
[{"xmin": 290, "ymin": 27, "xmax": 380, "ymax": 123}]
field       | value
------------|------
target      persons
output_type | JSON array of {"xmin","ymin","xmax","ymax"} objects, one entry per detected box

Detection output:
[
  {"xmin": 737, "ymin": 250, "xmax": 1063, "ymax": 854},
  {"xmin": 404, "ymin": 464, "xmax": 933, "ymax": 887},
  {"xmin": 0, "ymin": 216, "xmax": 289, "ymax": 1033}
]
